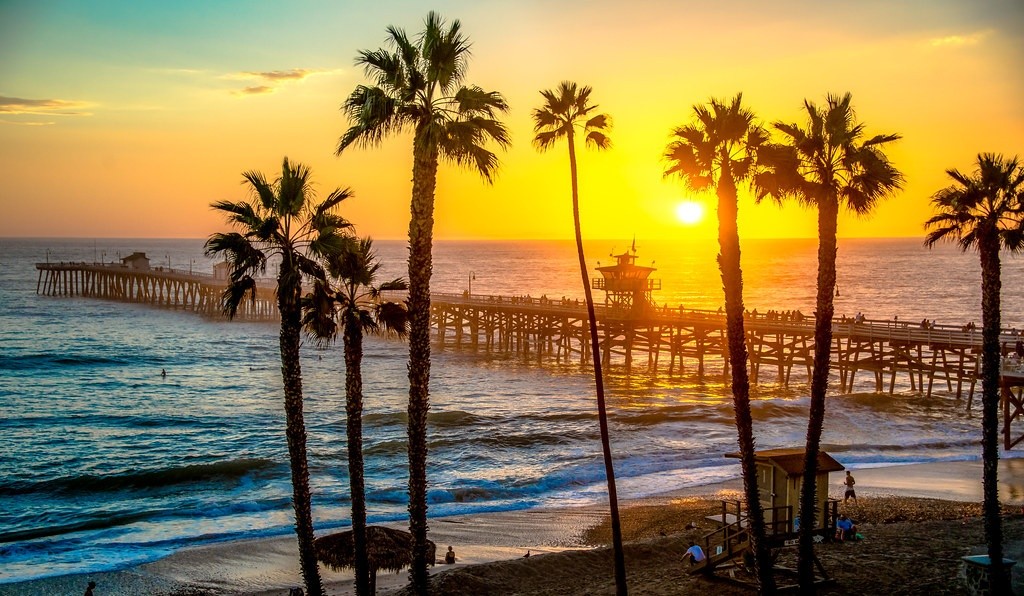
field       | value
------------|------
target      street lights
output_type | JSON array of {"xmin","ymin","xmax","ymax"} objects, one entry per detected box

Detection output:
[
  {"xmin": 46, "ymin": 247, "xmax": 51, "ymax": 262},
  {"xmin": 116, "ymin": 250, "xmax": 120, "ymax": 264},
  {"xmin": 102, "ymin": 249, "xmax": 106, "ymax": 265},
  {"xmin": 468, "ymin": 270, "xmax": 476, "ymax": 300},
  {"xmin": 190, "ymin": 256, "xmax": 196, "ymax": 276},
  {"xmin": 164, "ymin": 251, "xmax": 170, "ymax": 272}
]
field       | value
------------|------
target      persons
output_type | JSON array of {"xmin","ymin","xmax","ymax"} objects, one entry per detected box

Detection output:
[
  {"xmin": 718, "ymin": 306, "xmax": 723, "ymax": 315},
  {"xmin": 920, "ymin": 318, "xmax": 935, "ymax": 334},
  {"xmin": 856, "ymin": 312, "xmax": 867, "ymax": 326},
  {"xmin": 540, "ymin": 294, "xmax": 547, "ymax": 305},
  {"xmin": 462, "ymin": 290, "xmax": 532, "ymax": 305},
  {"xmin": 844, "ymin": 471, "xmax": 857, "ymax": 506},
  {"xmin": 679, "ymin": 304, "xmax": 685, "ymax": 317},
  {"xmin": 445, "ymin": 546, "xmax": 455, "ymax": 564},
  {"xmin": 744, "ymin": 308, "xmax": 802, "ymax": 325},
  {"xmin": 664, "ymin": 303, "xmax": 667, "ymax": 313},
  {"xmin": 680, "ymin": 542, "xmax": 706, "ymax": 567},
  {"xmin": 583, "ymin": 299, "xmax": 586, "ymax": 306},
  {"xmin": 1010, "ymin": 328, "xmax": 1018, "ymax": 340},
  {"xmin": 562, "ymin": 296, "xmax": 578, "ymax": 309},
  {"xmin": 962, "ymin": 322, "xmax": 975, "ymax": 340},
  {"xmin": 837, "ymin": 514, "xmax": 857, "ymax": 543}
]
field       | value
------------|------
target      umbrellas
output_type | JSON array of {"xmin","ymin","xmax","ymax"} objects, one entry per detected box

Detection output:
[{"xmin": 314, "ymin": 527, "xmax": 436, "ymax": 596}]
